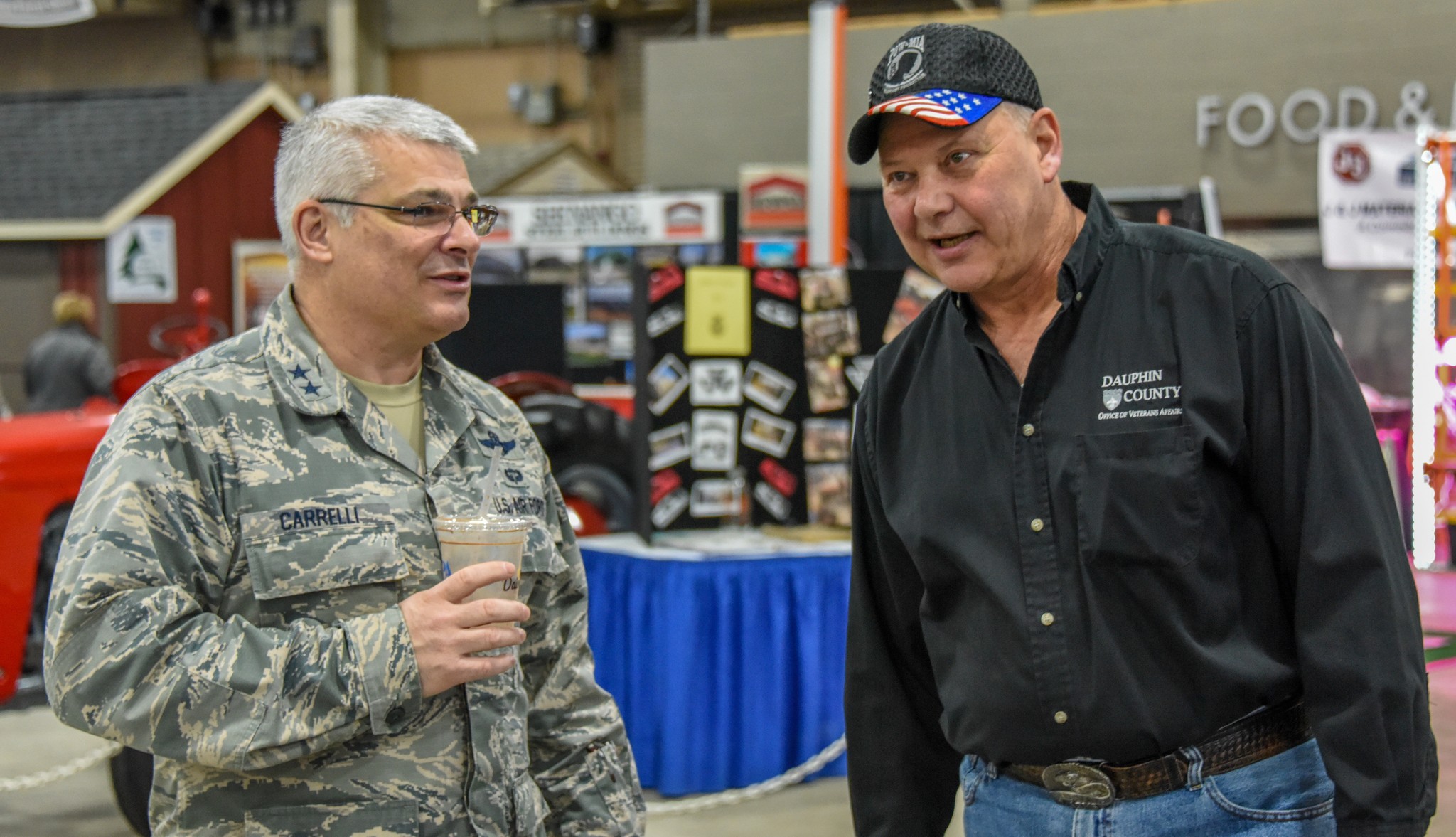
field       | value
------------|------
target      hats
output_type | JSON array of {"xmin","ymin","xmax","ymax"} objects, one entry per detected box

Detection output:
[
  {"xmin": 55, "ymin": 291, "xmax": 95, "ymax": 324},
  {"xmin": 848, "ymin": 21, "xmax": 1044, "ymax": 164}
]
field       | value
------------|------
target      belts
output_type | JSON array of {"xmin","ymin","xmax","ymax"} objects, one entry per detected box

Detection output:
[{"xmin": 987, "ymin": 699, "xmax": 1318, "ymax": 807}]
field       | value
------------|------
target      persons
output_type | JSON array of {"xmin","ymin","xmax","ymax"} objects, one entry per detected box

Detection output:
[
  {"xmin": 24, "ymin": 291, "xmax": 114, "ymax": 414},
  {"xmin": 42, "ymin": 95, "xmax": 646, "ymax": 837},
  {"xmin": 843, "ymin": 22, "xmax": 1440, "ymax": 837}
]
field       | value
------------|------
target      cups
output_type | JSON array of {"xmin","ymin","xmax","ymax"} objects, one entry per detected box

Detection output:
[{"xmin": 432, "ymin": 514, "xmax": 533, "ymax": 628}]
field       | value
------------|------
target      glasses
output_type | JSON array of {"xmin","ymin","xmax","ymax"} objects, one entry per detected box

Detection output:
[{"xmin": 317, "ymin": 199, "xmax": 499, "ymax": 237}]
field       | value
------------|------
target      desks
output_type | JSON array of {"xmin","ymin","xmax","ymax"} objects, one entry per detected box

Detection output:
[{"xmin": 561, "ymin": 521, "xmax": 877, "ymax": 792}]
